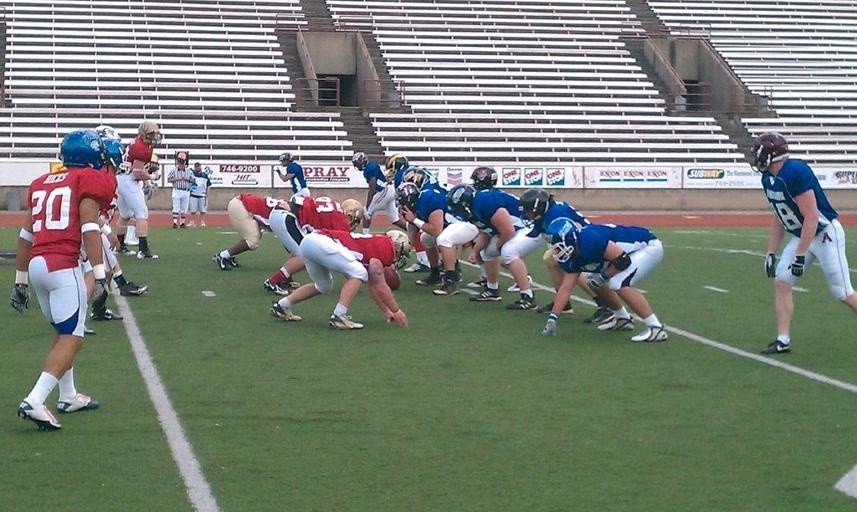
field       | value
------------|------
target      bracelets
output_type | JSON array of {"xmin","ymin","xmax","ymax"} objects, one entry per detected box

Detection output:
[
  {"xmin": 599, "ymin": 272, "xmax": 610, "ymax": 284},
  {"xmin": 413, "ymin": 218, "xmax": 425, "ymax": 229},
  {"xmin": 549, "ymin": 313, "xmax": 560, "ymax": 321},
  {"xmin": 393, "ymin": 308, "xmax": 401, "ymax": 315}
]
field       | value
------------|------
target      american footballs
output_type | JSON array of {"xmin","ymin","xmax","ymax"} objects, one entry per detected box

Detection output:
[{"xmin": 384, "ymin": 268, "xmax": 401, "ymax": 290}]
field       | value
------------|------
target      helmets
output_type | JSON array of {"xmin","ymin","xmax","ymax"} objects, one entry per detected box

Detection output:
[
  {"xmin": 470, "ymin": 167, "xmax": 497, "ymax": 190},
  {"xmin": 401, "ymin": 167, "xmax": 427, "ymax": 191},
  {"xmin": 545, "ymin": 216, "xmax": 580, "ymax": 264},
  {"xmin": 279, "ymin": 153, "xmax": 295, "ymax": 166},
  {"xmin": 352, "ymin": 152, "xmax": 368, "ymax": 171},
  {"xmin": 138, "ymin": 121, "xmax": 163, "ymax": 146},
  {"xmin": 749, "ymin": 133, "xmax": 789, "ymax": 172},
  {"xmin": 98, "ymin": 131, "xmax": 112, "ymax": 139},
  {"xmin": 384, "ymin": 153, "xmax": 409, "ymax": 180},
  {"xmin": 100, "ymin": 137, "xmax": 126, "ymax": 171},
  {"xmin": 396, "ymin": 183, "xmax": 420, "ymax": 217},
  {"xmin": 386, "ymin": 230, "xmax": 412, "ymax": 269},
  {"xmin": 341, "ymin": 199, "xmax": 364, "ymax": 230},
  {"xmin": 518, "ymin": 188, "xmax": 551, "ymax": 228},
  {"xmin": 95, "ymin": 126, "xmax": 122, "ymax": 140},
  {"xmin": 447, "ymin": 183, "xmax": 476, "ymax": 221},
  {"xmin": 61, "ymin": 129, "xmax": 107, "ymax": 169},
  {"xmin": 144, "ymin": 154, "xmax": 159, "ymax": 173}
]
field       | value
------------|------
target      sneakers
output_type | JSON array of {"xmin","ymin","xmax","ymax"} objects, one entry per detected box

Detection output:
[
  {"xmin": 631, "ymin": 324, "xmax": 668, "ymax": 342},
  {"xmin": 433, "ymin": 280, "xmax": 460, "ymax": 295},
  {"xmin": 137, "ymin": 250, "xmax": 159, "ymax": 259},
  {"xmin": 434, "ymin": 271, "xmax": 463, "ymax": 285},
  {"xmin": 507, "ymin": 274, "xmax": 533, "ymax": 291},
  {"xmin": 584, "ymin": 306, "xmax": 613, "ymax": 323},
  {"xmin": 415, "ymin": 274, "xmax": 444, "ymax": 287},
  {"xmin": 271, "ymin": 303, "xmax": 301, "ymax": 321},
  {"xmin": 85, "ymin": 325, "xmax": 94, "ymax": 335},
  {"xmin": 404, "ymin": 262, "xmax": 431, "ymax": 273},
  {"xmin": 212, "ymin": 252, "xmax": 231, "ymax": 272},
  {"xmin": 761, "ymin": 341, "xmax": 790, "ymax": 354},
  {"xmin": 228, "ymin": 256, "xmax": 238, "ymax": 267},
  {"xmin": 506, "ymin": 292, "xmax": 538, "ymax": 309},
  {"xmin": 329, "ymin": 312, "xmax": 364, "ymax": 329},
  {"xmin": 469, "ymin": 285, "xmax": 502, "ymax": 301},
  {"xmin": 439, "ymin": 258, "xmax": 458, "ymax": 266},
  {"xmin": 467, "ymin": 274, "xmax": 487, "ymax": 288},
  {"xmin": 114, "ymin": 247, "xmax": 136, "ymax": 256},
  {"xmin": 263, "ymin": 279, "xmax": 289, "ymax": 295},
  {"xmin": 90, "ymin": 309, "xmax": 123, "ymax": 320},
  {"xmin": 540, "ymin": 300, "xmax": 574, "ymax": 314},
  {"xmin": 58, "ymin": 394, "xmax": 100, "ymax": 412},
  {"xmin": 278, "ymin": 281, "xmax": 301, "ymax": 289},
  {"xmin": 17, "ymin": 398, "xmax": 62, "ymax": 431},
  {"xmin": 598, "ymin": 314, "xmax": 635, "ymax": 330},
  {"xmin": 120, "ymin": 281, "xmax": 148, "ymax": 296}
]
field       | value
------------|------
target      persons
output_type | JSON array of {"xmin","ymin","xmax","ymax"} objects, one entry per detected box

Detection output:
[
  {"xmin": 9, "ymin": 130, "xmax": 113, "ymax": 431},
  {"xmin": 402, "ymin": 167, "xmax": 464, "ymax": 275},
  {"xmin": 269, "ymin": 228, "xmax": 412, "ymax": 329},
  {"xmin": 541, "ymin": 217, "xmax": 668, "ymax": 342},
  {"xmin": 167, "ymin": 152, "xmax": 195, "ymax": 228},
  {"xmin": 48, "ymin": 122, "xmax": 161, "ymax": 335},
  {"xmin": 518, "ymin": 188, "xmax": 615, "ymax": 324},
  {"xmin": 445, "ymin": 184, "xmax": 546, "ymax": 310},
  {"xmin": 186, "ymin": 162, "xmax": 212, "ymax": 227},
  {"xmin": 276, "ymin": 153, "xmax": 310, "ymax": 201},
  {"xmin": 467, "ymin": 166, "xmax": 532, "ymax": 293},
  {"xmin": 212, "ymin": 193, "xmax": 279, "ymax": 272},
  {"xmin": 352, "ymin": 152, "xmax": 408, "ymax": 233},
  {"xmin": 384, "ymin": 154, "xmax": 439, "ymax": 251},
  {"xmin": 263, "ymin": 194, "xmax": 365, "ymax": 296},
  {"xmin": 750, "ymin": 133, "xmax": 857, "ymax": 354},
  {"xmin": 396, "ymin": 181, "xmax": 479, "ymax": 295}
]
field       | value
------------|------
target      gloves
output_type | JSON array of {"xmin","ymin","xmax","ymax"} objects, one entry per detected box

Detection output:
[
  {"xmin": 10, "ymin": 285, "xmax": 30, "ymax": 313},
  {"xmin": 788, "ymin": 256, "xmax": 805, "ymax": 277},
  {"xmin": 91, "ymin": 279, "xmax": 109, "ymax": 309},
  {"xmin": 541, "ymin": 314, "xmax": 558, "ymax": 335},
  {"xmin": 585, "ymin": 272, "xmax": 609, "ymax": 292},
  {"xmin": 764, "ymin": 253, "xmax": 775, "ymax": 278},
  {"xmin": 149, "ymin": 171, "xmax": 161, "ymax": 181}
]
